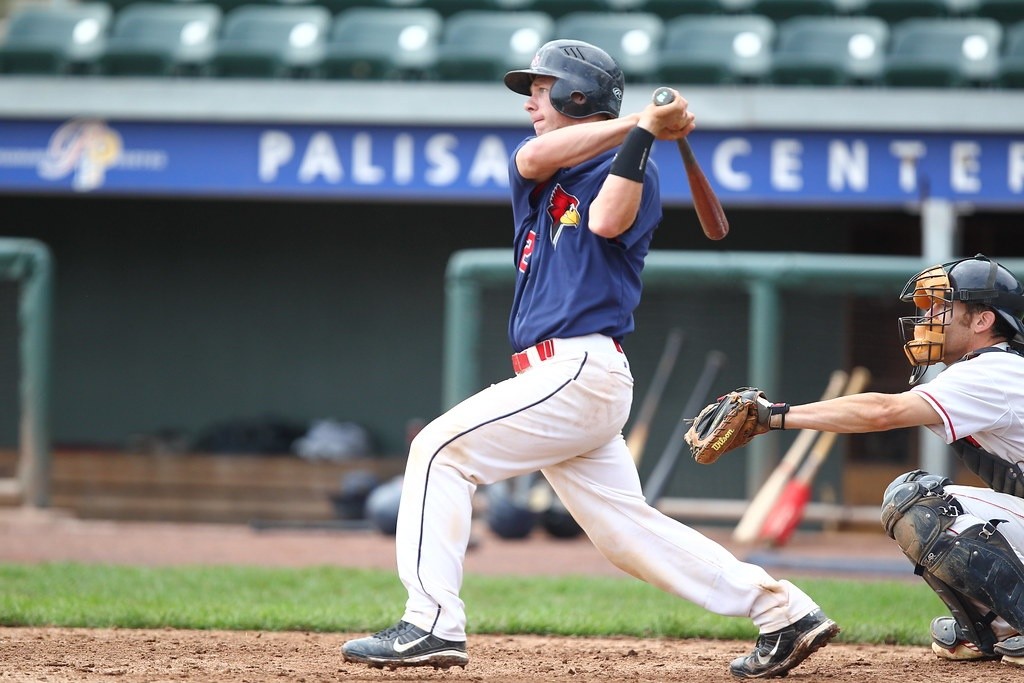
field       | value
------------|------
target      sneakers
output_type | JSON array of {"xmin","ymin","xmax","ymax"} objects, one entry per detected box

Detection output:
[
  {"xmin": 341, "ymin": 619, "xmax": 468, "ymax": 665},
  {"xmin": 729, "ymin": 607, "xmax": 838, "ymax": 679}
]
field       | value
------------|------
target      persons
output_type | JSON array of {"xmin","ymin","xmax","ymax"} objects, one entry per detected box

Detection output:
[
  {"xmin": 340, "ymin": 38, "xmax": 841, "ymax": 679},
  {"xmin": 682, "ymin": 254, "xmax": 1024, "ymax": 669}
]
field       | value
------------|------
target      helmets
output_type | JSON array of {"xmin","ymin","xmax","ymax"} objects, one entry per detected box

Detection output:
[
  {"xmin": 948, "ymin": 260, "xmax": 1024, "ymax": 336},
  {"xmin": 504, "ymin": 40, "xmax": 624, "ymax": 119}
]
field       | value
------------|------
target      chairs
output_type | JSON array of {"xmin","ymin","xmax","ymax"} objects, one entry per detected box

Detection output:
[
  {"xmin": 188, "ymin": 3, "xmax": 332, "ymax": 77},
  {"xmin": 648, "ymin": 13, "xmax": 778, "ymax": 85},
  {"xmin": 992, "ymin": 21, "xmax": 1024, "ymax": 87},
  {"xmin": 100, "ymin": 2, "xmax": 224, "ymax": 76},
  {"xmin": 769, "ymin": 14, "xmax": 893, "ymax": 83},
  {"xmin": 547, "ymin": 11, "xmax": 666, "ymax": 82},
  {"xmin": 0, "ymin": 3, "xmax": 113, "ymax": 74},
  {"xmin": 885, "ymin": 17, "xmax": 1004, "ymax": 85},
  {"xmin": 432, "ymin": 8, "xmax": 555, "ymax": 82},
  {"xmin": 304, "ymin": 6, "xmax": 444, "ymax": 80}
]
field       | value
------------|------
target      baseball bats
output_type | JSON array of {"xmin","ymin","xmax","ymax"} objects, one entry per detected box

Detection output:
[
  {"xmin": 728, "ymin": 364, "xmax": 869, "ymax": 550},
  {"xmin": 625, "ymin": 325, "xmax": 727, "ymax": 509},
  {"xmin": 653, "ymin": 87, "xmax": 730, "ymax": 242}
]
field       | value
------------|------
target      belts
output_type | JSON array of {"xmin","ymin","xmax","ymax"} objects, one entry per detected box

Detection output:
[{"xmin": 511, "ymin": 334, "xmax": 624, "ymax": 371}]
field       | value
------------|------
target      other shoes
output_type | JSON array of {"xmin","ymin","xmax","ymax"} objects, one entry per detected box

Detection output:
[{"xmin": 930, "ymin": 616, "xmax": 1024, "ymax": 667}]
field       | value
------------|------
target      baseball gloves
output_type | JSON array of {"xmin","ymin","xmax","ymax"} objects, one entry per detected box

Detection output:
[{"xmin": 682, "ymin": 386, "xmax": 786, "ymax": 464}]
